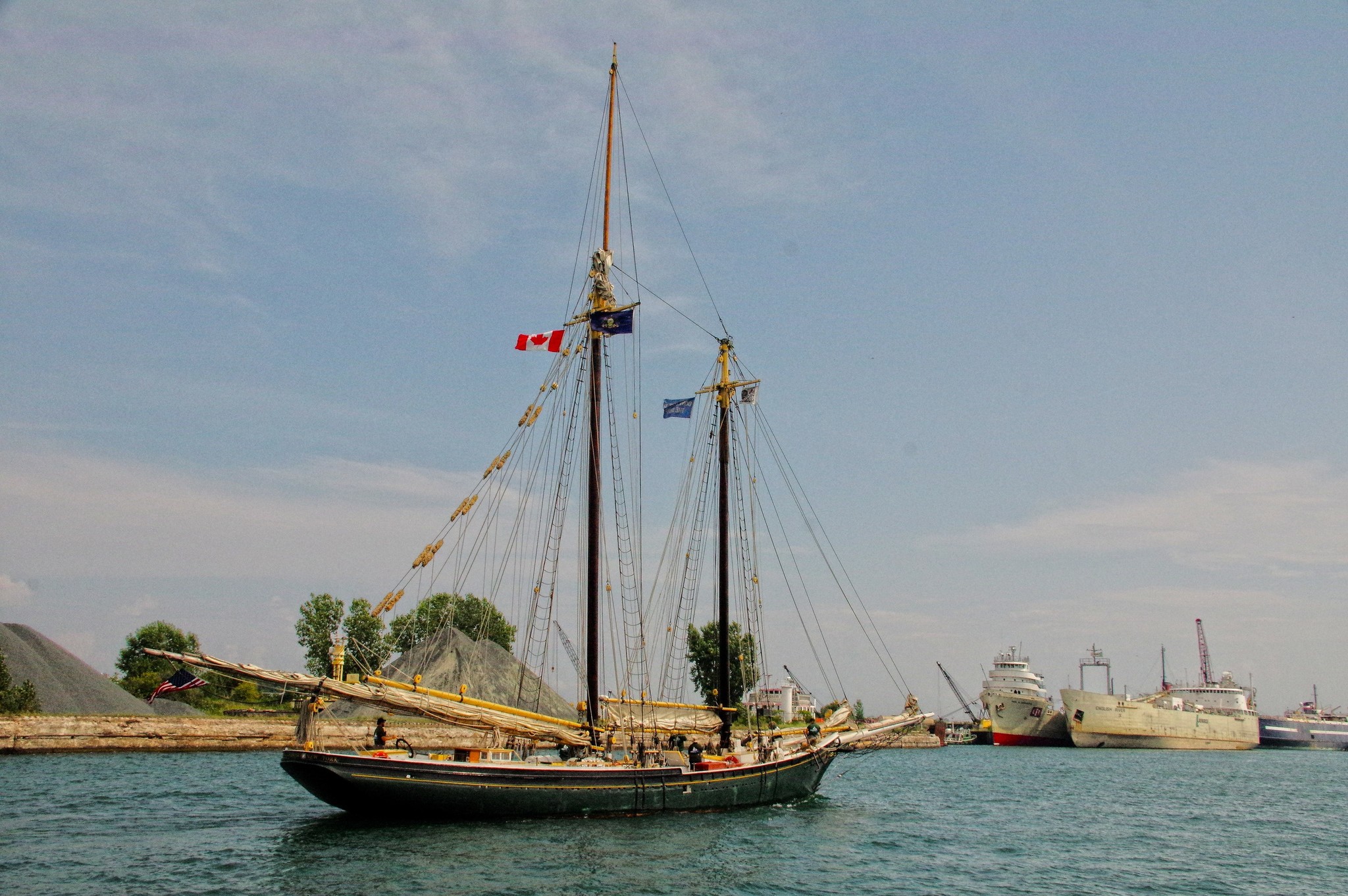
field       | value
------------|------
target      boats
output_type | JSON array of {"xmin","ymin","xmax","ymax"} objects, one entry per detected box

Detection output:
[
  {"xmin": 1066, "ymin": 618, "xmax": 1261, "ymax": 750},
  {"xmin": 979, "ymin": 641, "xmax": 1074, "ymax": 747},
  {"xmin": 943, "ymin": 729, "xmax": 977, "ymax": 745},
  {"xmin": 1259, "ymin": 685, "xmax": 1348, "ymax": 749}
]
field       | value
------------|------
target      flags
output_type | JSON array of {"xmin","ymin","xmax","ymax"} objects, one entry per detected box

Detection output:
[
  {"xmin": 660, "ymin": 396, "xmax": 695, "ymax": 418},
  {"xmin": 147, "ymin": 668, "xmax": 208, "ymax": 705},
  {"xmin": 514, "ymin": 327, "xmax": 565, "ymax": 353},
  {"xmin": 592, "ymin": 310, "xmax": 633, "ymax": 336},
  {"xmin": 739, "ymin": 386, "xmax": 759, "ymax": 405}
]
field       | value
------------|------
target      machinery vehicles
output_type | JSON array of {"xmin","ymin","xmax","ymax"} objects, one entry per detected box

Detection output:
[{"xmin": 936, "ymin": 660, "xmax": 991, "ymax": 732}]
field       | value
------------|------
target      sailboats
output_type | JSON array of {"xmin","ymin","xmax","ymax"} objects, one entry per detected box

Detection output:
[{"xmin": 137, "ymin": 38, "xmax": 934, "ymax": 823}]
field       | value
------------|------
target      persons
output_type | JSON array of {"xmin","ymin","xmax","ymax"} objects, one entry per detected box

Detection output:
[
  {"xmin": 637, "ymin": 738, "xmax": 646, "ymax": 766},
  {"xmin": 946, "ymin": 726, "xmax": 951, "ymax": 733},
  {"xmin": 704, "ymin": 744, "xmax": 707, "ymax": 750},
  {"xmin": 770, "ymin": 741, "xmax": 785, "ymax": 761},
  {"xmin": 688, "ymin": 737, "xmax": 703, "ymax": 772},
  {"xmin": 805, "ymin": 718, "xmax": 822, "ymax": 738},
  {"xmin": 668, "ymin": 733, "xmax": 687, "ymax": 753},
  {"xmin": 768, "ymin": 721, "xmax": 792, "ymax": 751},
  {"xmin": 373, "ymin": 717, "xmax": 398, "ymax": 750},
  {"xmin": 654, "ymin": 734, "xmax": 660, "ymax": 745}
]
